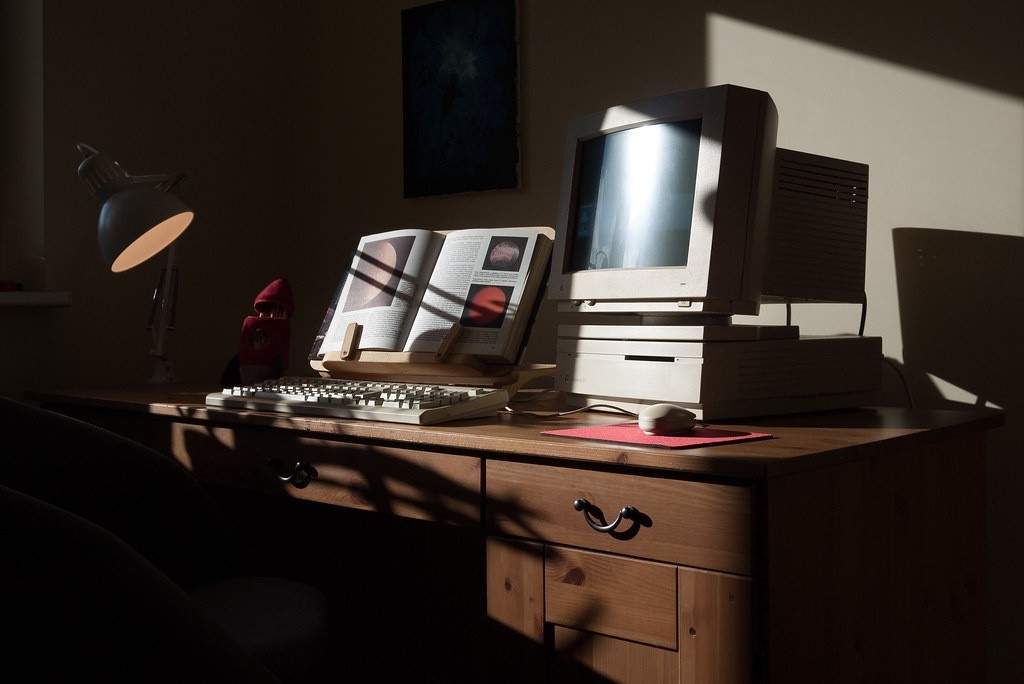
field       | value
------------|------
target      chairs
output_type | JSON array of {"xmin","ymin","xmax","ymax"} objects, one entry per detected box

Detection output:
[{"xmin": 0, "ymin": 395, "xmax": 333, "ymax": 684}]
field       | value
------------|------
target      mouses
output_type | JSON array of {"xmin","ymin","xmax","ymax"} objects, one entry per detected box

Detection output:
[{"xmin": 638, "ymin": 403, "xmax": 697, "ymax": 435}]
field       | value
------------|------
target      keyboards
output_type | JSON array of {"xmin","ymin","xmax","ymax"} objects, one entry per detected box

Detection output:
[{"xmin": 206, "ymin": 376, "xmax": 509, "ymax": 426}]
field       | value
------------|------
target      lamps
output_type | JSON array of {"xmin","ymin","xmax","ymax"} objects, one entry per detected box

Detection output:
[{"xmin": 76, "ymin": 142, "xmax": 195, "ymax": 376}]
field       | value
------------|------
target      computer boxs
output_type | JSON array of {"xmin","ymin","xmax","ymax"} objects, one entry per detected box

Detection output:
[{"xmin": 553, "ymin": 334, "xmax": 883, "ymax": 421}]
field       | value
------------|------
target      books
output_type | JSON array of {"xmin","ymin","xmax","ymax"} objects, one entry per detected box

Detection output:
[{"xmin": 317, "ymin": 227, "xmax": 557, "ymax": 366}]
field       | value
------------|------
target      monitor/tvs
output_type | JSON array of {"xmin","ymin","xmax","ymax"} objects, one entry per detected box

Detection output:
[{"xmin": 548, "ymin": 83, "xmax": 867, "ymax": 321}]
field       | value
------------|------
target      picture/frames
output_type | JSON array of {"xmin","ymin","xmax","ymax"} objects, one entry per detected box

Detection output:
[{"xmin": 401, "ymin": 0, "xmax": 529, "ymax": 202}]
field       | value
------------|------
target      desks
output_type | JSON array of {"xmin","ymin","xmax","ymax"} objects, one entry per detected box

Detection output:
[{"xmin": 23, "ymin": 378, "xmax": 1006, "ymax": 684}]
